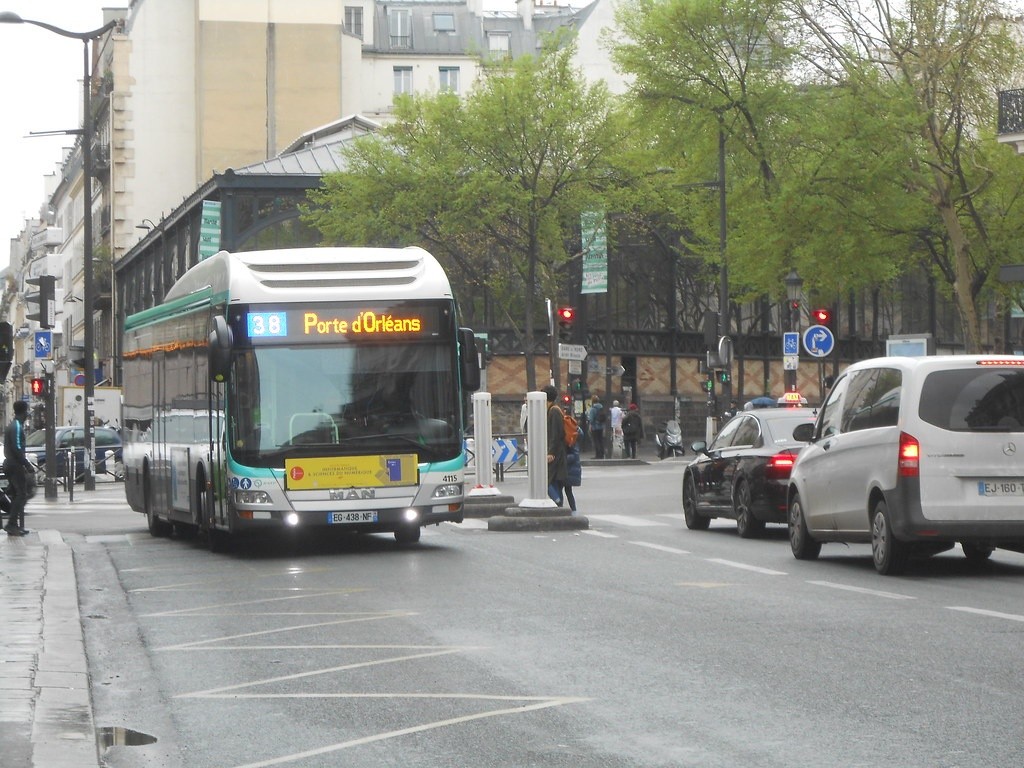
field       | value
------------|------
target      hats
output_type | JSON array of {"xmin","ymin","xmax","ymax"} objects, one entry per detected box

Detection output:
[{"xmin": 630, "ymin": 404, "xmax": 637, "ymax": 409}]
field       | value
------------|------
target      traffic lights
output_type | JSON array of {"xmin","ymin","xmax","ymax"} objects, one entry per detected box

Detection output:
[
  {"xmin": 563, "ymin": 395, "xmax": 571, "ymax": 403},
  {"xmin": 699, "ymin": 382, "xmax": 708, "ymax": 392},
  {"xmin": 25, "ymin": 274, "xmax": 55, "ymax": 328},
  {"xmin": 699, "ymin": 360, "xmax": 711, "ymax": 375},
  {"xmin": 30, "ymin": 378, "xmax": 42, "ymax": 396},
  {"xmin": 812, "ymin": 309, "xmax": 832, "ymax": 325},
  {"xmin": 557, "ymin": 307, "xmax": 577, "ymax": 342},
  {"xmin": 716, "ymin": 370, "xmax": 730, "ymax": 384},
  {"xmin": 707, "ymin": 350, "xmax": 720, "ymax": 370}
]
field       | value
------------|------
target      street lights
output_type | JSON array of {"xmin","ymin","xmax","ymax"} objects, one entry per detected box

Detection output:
[
  {"xmin": 632, "ymin": 88, "xmax": 733, "ymax": 428},
  {"xmin": 0, "ymin": 10, "xmax": 96, "ymax": 492}
]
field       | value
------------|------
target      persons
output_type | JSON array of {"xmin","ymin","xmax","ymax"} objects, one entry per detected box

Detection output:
[
  {"xmin": 465, "ymin": 414, "xmax": 474, "ymax": 434},
  {"xmin": 725, "ymin": 402, "xmax": 737, "ymax": 417},
  {"xmin": 589, "ymin": 395, "xmax": 606, "ymax": 459},
  {"xmin": 622, "ymin": 403, "xmax": 644, "ymax": 458},
  {"xmin": 520, "ymin": 394, "xmax": 528, "ymax": 433},
  {"xmin": 540, "ymin": 385, "xmax": 585, "ymax": 515},
  {"xmin": 4, "ymin": 401, "xmax": 35, "ymax": 536},
  {"xmin": 610, "ymin": 400, "xmax": 627, "ymax": 449}
]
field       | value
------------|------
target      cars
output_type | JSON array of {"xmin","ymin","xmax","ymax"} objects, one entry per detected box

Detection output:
[
  {"xmin": 681, "ymin": 407, "xmax": 821, "ymax": 538},
  {"xmin": 0, "ymin": 425, "xmax": 126, "ymax": 488}
]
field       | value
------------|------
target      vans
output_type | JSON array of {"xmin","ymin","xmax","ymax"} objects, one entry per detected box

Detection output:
[{"xmin": 786, "ymin": 355, "xmax": 1024, "ymax": 576}]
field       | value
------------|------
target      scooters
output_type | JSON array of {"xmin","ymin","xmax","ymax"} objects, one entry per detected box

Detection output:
[{"xmin": 654, "ymin": 418, "xmax": 686, "ymax": 459}]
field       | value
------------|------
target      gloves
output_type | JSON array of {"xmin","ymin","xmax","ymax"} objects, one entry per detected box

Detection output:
[{"xmin": 25, "ymin": 462, "xmax": 36, "ymax": 474}]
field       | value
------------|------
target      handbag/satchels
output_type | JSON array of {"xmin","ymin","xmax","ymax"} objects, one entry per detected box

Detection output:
[{"xmin": 621, "ymin": 416, "xmax": 636, "ymax": 435}]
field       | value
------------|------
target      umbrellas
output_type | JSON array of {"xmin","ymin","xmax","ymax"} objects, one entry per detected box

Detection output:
[{"xmin": 749, "ymin": 397, "xmax": 776, "ymax": 405}]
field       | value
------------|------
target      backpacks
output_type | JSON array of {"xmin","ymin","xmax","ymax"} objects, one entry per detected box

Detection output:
[
  {"xmin": 549, "ymin": 406, "xmax": 579, "ymax": 447},
  {"xmin": 595, "ymin": 408, "xmax": 607, "ymax": 425}
]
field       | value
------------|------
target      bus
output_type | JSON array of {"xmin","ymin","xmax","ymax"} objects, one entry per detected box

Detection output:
[{"xmin": 121, "ymin": 246, "xmax": 482, "ymax": 552}]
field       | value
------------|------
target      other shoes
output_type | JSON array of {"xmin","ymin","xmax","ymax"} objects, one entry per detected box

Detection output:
[{"xmin": 3, "ymin": 524, "xmax": 30, "ymax": 535}]
